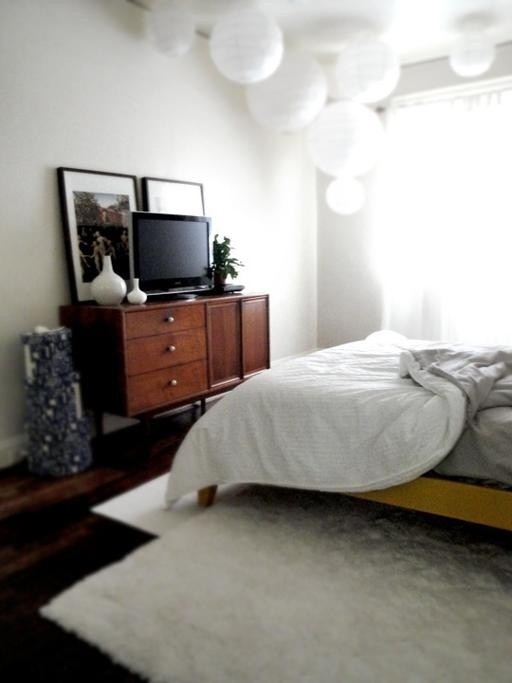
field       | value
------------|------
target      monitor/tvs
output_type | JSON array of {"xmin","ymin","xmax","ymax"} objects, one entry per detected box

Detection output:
[{"xmin": 128, "ymin": 211, "xmax": 212, "ymax": 305}]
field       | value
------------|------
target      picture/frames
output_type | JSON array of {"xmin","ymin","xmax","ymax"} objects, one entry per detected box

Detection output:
[
  {"xmin": 56, "ymin": 167, "xmax": 140, "ymax": 306},
  {"xmin": 141, "ymin": 177, "xmax": 212, "ymax": 296}
]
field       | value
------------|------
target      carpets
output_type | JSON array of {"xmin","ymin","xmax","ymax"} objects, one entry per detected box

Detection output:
[
  {"xmin": 39, "ymin": 484, "xmax": 511, "ymax": 683},
  {"xmin": 89, "ymin": 469, "xmax": 244, "ymax": 536}
]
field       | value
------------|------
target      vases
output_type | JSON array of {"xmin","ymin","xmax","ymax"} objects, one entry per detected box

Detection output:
[
  {"xmin": 90, "ymin": 252, "xmax": 127, "ymax": 305},
  {"xmin": 127, "ymin": 278, "xmax": 148, "ymax": 304}
]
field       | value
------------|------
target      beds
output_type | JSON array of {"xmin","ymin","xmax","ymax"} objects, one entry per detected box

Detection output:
[{"xmin": 162, "ymin": 338, "xmax": 512, "ymax": 532}]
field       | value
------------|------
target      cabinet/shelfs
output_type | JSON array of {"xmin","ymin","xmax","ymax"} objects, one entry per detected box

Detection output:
[{"xmin": 59, "ymin": 291, "xmax": 270, "ymax": 418}]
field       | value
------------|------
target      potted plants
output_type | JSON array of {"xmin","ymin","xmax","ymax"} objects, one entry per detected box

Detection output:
[{"xmin": 210, "ymin": 234, "xmax": 244, "ymax": 286}]
